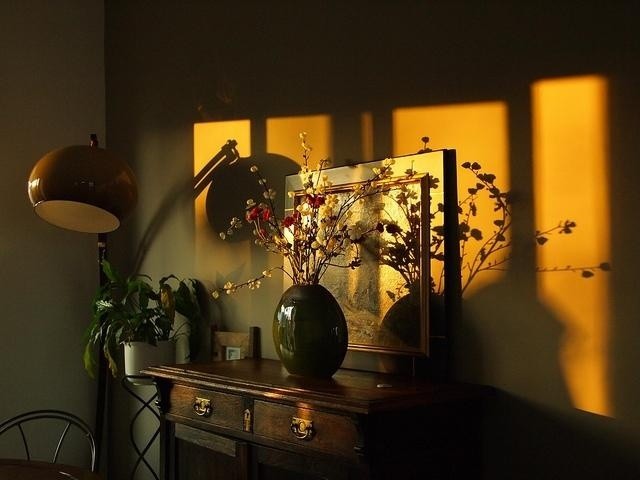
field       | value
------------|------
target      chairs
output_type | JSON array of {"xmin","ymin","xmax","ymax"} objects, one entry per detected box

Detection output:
[{"xmin": 1, "ymin": 410, "xmax": 107, "ymax": 462}]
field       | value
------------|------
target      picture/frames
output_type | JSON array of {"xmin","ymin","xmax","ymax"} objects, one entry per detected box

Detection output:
[{"xmin": 281, "ymin": 150, "xmax": 448, "ymax": 362}]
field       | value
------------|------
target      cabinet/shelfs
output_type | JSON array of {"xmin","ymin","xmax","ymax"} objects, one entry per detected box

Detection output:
[{"xmin": 141, "ymin": 360, "xmax": 495, "ymax": 480}]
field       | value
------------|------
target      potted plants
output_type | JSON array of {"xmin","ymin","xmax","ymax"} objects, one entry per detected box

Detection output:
[{"xmin": 81, "ymin": 260, "xmax": 198, "ymax": 388}]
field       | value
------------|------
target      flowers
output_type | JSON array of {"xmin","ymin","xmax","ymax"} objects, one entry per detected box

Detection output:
[{"xmin": 214, "ymin": 137, "xmax": 410, "ymax": 283}]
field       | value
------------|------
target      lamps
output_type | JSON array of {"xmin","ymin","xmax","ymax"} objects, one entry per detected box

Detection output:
[{"xmin": 24, "ymin": 137, "xmax": 143, "ymax": 475}]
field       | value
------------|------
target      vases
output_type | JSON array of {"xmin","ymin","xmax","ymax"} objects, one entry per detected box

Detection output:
[{"xmin": 272, "ymin": 285, "xmax": 348, "ymax": 382}]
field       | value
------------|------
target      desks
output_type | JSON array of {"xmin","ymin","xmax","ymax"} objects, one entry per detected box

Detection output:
[{"xmin": 0, "ymin": 465, "xmax": 100, "ymax": 480}]
